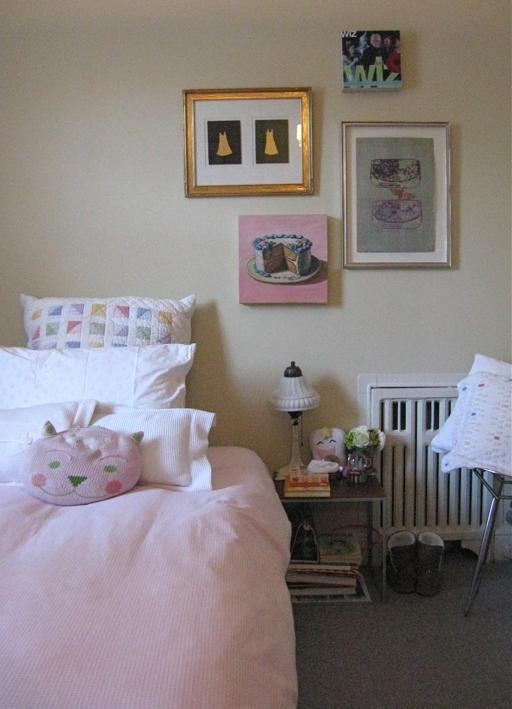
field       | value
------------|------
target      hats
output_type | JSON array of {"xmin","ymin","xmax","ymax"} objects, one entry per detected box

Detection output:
[
  {"xmin": 359, "ymin": 31, "xmax": 367, "ymax": 42},
  {"xmin": 371, "ymin": 34, "xmax": 381, "ymax": 41}
]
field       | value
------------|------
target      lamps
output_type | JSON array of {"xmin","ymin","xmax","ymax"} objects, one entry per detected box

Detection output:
[{"xmin": 269, "ymin": 361, "xmax": 320, "ymax": 481}]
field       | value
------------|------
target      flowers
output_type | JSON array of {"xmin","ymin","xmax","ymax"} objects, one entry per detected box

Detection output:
[{"xmin": 342, "ymin": 426, "xmax": 387, "ymax": 452}]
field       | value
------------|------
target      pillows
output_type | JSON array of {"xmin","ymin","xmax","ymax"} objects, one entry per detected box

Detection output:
[
  {"xmin": 443, "ymin": 372, "xmax": 511, "ymax": 473},
  {"xmin": 428, "ymin": 355, "xmax": 512, "ymax": 453},
  {"xmin": 19, "ymin": 293, "xmax": 194, "ymax": 346},
  {"xmin": 1, "ymin": 344, "xmax": 194, "ymax": 406},
  {"xmin": 2, "ymin": 401, "xmax": 97, "ymax": 481},
  {"xmin": 19, "ymin": 420, "xmax": 144, "ymax": 505},
  {"xmin": 85, "ymin": 408, "xmax": 217, "ymax": 493}
]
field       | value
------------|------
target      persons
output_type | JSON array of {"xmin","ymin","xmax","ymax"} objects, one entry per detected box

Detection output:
[{"xmin": 342, "ymin": 34, "xmax": 400, "ymax": 87}]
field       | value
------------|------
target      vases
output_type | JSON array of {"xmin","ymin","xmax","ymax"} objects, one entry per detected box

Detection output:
[{"xmin": 344, "ymin": 453, "xmax": 376, "ymax": 490}]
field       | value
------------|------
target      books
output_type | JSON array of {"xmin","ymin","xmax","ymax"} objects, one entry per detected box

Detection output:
[
  {"xmin": 283, "ymin": 472, "xmax": 331, "ymax": 498},
  {"xmin": 285, "ymin": 530, "xmax": 361, "ymax": 596}
]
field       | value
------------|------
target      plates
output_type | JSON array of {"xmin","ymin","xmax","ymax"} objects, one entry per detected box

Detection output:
[{"xmin": 245, "ymin": 253, "xmax": 322, "ymax": 283}]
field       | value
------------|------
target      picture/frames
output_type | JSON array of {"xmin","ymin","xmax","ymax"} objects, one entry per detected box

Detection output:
[
  {"xmin": 341, "ymin": 121, "xmax": 450, "ymax": 268},
  {"xmin": 237, "ymin": 214, "xmax": 330, "ymax": 306},
  {"xmin": 182, "ymin": 86, "xmax": 316, "ymax": 196},
  {"xmin": 338, "ymin": 28, "xmax": 405, "ymax": 93}
]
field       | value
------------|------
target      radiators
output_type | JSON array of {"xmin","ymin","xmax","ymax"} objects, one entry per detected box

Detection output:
[{"xmin": 359, "ymin": 372, "xmax": 509, "ymax": 569}]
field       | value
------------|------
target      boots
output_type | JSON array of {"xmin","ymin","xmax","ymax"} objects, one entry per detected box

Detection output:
[
  {"xmin": 416, "ymin": 530, "xmax": 445, "ymax": 597},
  {"xmin": 379, "ymin": 527, "xmax": 416, "ymax": 595}
]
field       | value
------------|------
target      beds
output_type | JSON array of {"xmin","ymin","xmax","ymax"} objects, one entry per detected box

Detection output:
[{"xmin": 2, "ymin": 446, "xmax": 299, "ymax": 709}]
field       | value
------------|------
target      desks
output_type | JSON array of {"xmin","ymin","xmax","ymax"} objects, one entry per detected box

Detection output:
[{"xmin": 280, "ymin": 476, "xmax": 389, "ymax": 603}]
